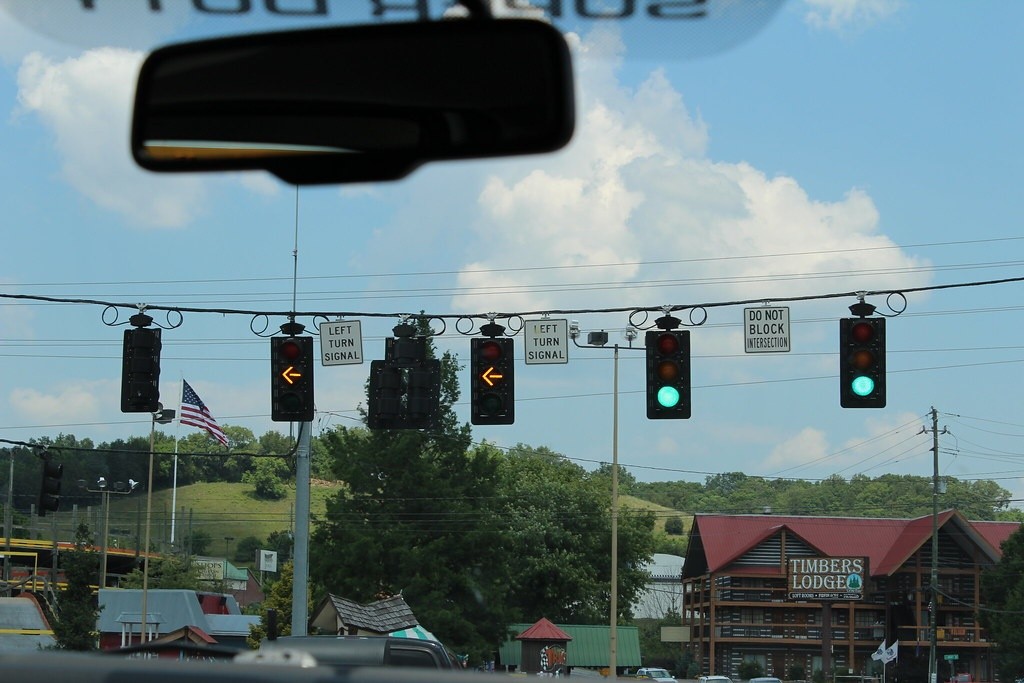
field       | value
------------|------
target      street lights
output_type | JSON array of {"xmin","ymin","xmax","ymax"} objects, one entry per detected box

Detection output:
[
  {"xmin": 141, "ymin": 409, "xmax": 177, "ymax": 659},
  {"xmin": 78, "ymin": 477, "xmax": 139, "ymax": 589},
  {"xmin": 573, "ymin": 332, "xmax": 645, "ymax": 678},
  {"xmin": 224, "ymin": 537, "xmax": 234, "ymax": 578},
  {"xmin": 2, "ymin": 446, "xmax": 21, "ymax": 581}
]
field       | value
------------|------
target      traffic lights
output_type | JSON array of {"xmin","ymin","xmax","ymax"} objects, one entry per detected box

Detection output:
[
  {"xmin": 271, "ymin": 337, "xmax": 314, "ymax": 421},
  {"xmin": 471, "ymin": 338, "xmax": 514, "ymax": 425},
  {"xmin": 839, "ymin": 317, "xmax": 887, "ymax": 409},
  {"xmin": 37, "ymin": 460, "xmax": 63, "ymax": 516},
  {"xmin": 122, "ymin": 328, "xmax": 162, "ymax": 413},
  {"xmin": 646, "ymin": 330, "xmax": 691, "ymax": 420}
]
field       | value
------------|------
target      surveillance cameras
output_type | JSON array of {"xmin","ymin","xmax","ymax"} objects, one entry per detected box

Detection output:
[
  {"xmin": 624, "ymin": 326, "xmax": 638, "ymax": 339},
  {"xmin": 569, "ymin": 320, "xmax": 581, "ymax": 338}
]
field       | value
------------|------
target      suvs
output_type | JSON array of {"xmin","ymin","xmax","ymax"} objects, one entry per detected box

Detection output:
[{"xmin": 637, "ymin": 667, "xmax": 678, "ymax": 683}]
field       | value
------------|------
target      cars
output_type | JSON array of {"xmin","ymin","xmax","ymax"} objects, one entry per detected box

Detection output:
[
  {"xmin": 260, "ymin": 636, "xmax": 465, "ymax": 670},
  {"xmin": 698, "ymin": 675, "xmax": 734, "ymax": 683},
  {"xmin": 749, "ymin": 677, "xmax": 782, "ymax": 683}
]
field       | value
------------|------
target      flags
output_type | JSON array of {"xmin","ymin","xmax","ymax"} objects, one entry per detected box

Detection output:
[
  {"xmin": 180, "ymin": 379, "xmax": 229, "ymax": 449},
  {"xmin": 871, "ymin": 641, "xmax": 885, "ymax": 661},
  {"xmin": 880, "ymin": 641, "xmax": 897, "ymax": 664}
]
